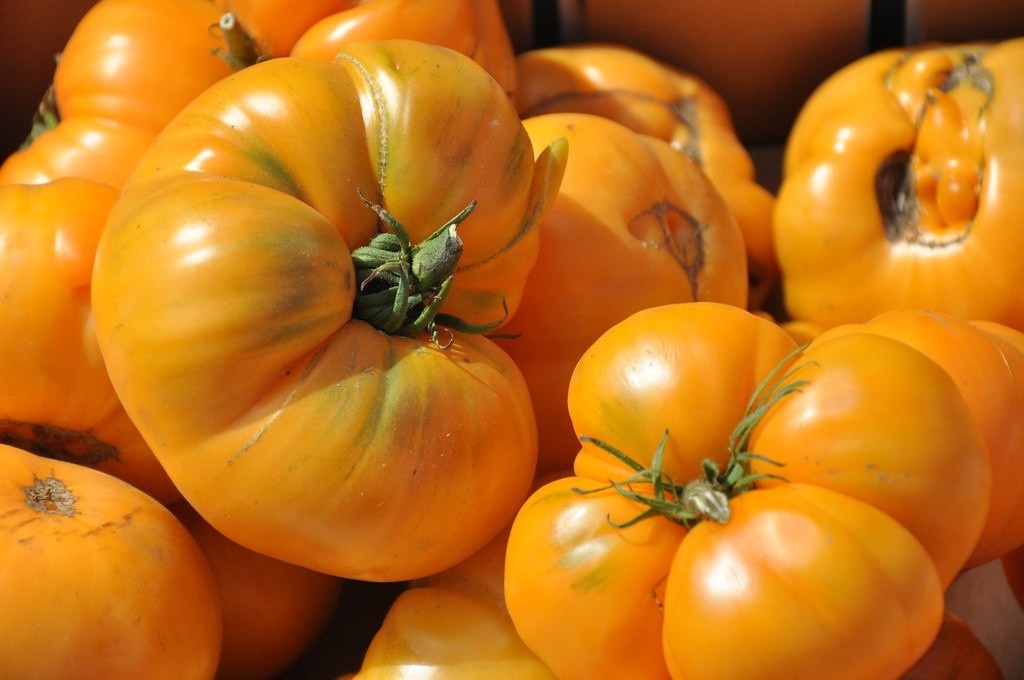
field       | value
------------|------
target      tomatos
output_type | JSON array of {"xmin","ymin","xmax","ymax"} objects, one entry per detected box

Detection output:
[{"xmin": 0, "ymin": 0, "xmax": 1024, "ymax": 680}]
